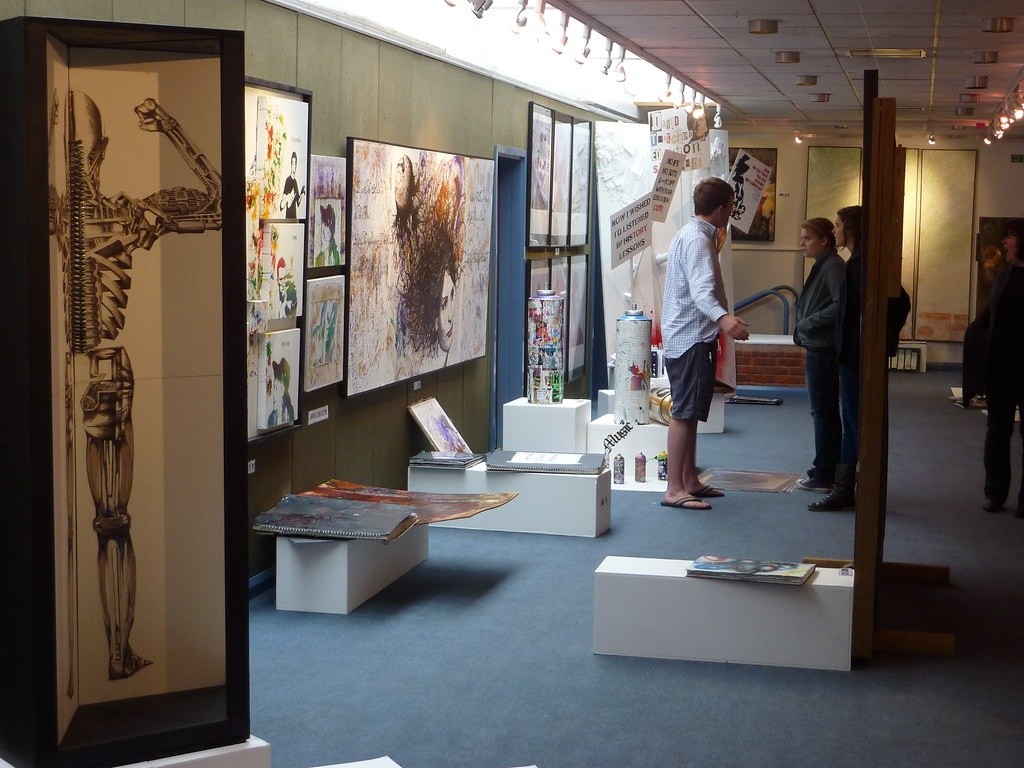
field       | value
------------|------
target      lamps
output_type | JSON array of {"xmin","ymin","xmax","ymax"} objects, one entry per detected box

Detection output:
[
  {"xmin": 928, "ymin": 129, "xmax": 935, "ymax": 144},
  {"xmin": 794, "ymin": 131, "xmax": 803, "ymax": 143},
  {"xmin": 984, "ymin": 80, "xmax": 1024, "ymax": 144},
  {"xmin": 749, "ymin": 19, "xmax": 847, "ymax": 129},
  {"xmin": 953, "ymin": 17, "xmax": 1014, "ymax": 130},
  {"xmin": 445, "ymin": 0, "xmax": 723, "ymax": 129}
]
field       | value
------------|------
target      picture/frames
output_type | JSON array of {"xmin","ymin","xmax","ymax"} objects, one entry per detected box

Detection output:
[{"xmin": 729, "ymin": 147, "xmax": 778, "ymax": 243}]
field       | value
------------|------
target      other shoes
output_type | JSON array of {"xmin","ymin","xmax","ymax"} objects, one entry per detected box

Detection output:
[
  {"xmin": 954, "ymin": 402, "xmax": 968, "ymax": 410},
  {"xmin": 983, "ymin": 498, "xmax": 1004, "ymax": 512},
  {"xmin": 1013, "ymin": 507, "xmax": 1024, "ymax": 518}
]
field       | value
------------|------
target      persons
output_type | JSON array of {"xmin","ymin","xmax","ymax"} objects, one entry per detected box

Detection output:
[
  {"xmin": 47, "ymin": 89, "xmax": 223, "ymax": 695},
  {"xmin": 792, "ymin": 218, "xmax": 845, "ymax": 492},
  {"xmin": 660, "ymin": 177, "xmax": 751, "ymax": 510},
  {"xmin": 962, "ymin": 219, "xmax": 1024, "ymax": 517},
  {"xmin": 688, "ymin": 105, "xmax": 710, "ymax": 143},
  {"xmin": 806, "ymin": 206, "xmax": 911, "ymax": 512}
]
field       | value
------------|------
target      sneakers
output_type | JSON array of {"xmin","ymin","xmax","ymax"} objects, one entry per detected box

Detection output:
[{"xmin": 796, "ymin": 478, "xmax": 834, "ymax": 491}]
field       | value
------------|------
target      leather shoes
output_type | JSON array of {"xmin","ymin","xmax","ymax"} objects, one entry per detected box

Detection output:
[{"xmin": 807, "ymin": 489, "xmax": 855, "ymax": 512}]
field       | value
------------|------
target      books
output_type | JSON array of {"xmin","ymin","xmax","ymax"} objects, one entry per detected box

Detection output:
[
  {"xmin": 251, "ymin": 494, "xmax": 422, "ymax": 543},
  {"xmin": 685, "ymin": 555, "xmax": 816, "ymax": 586},
  {"xmin": 487, "ymin": 447, "xmax": 607, "ymax": 476},
  {"xmin": 409, "ymin": 451, "xmax": 485, "ymax": 470}
]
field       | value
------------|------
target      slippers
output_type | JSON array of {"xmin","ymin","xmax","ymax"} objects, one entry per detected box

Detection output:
[
  {"xmin": 660, "ymin": 497, "xmax": 713, "ymax": 510},
  {"xmin": 690, "ymin": 486, "xmax": 724, "ymax": 496}
]
